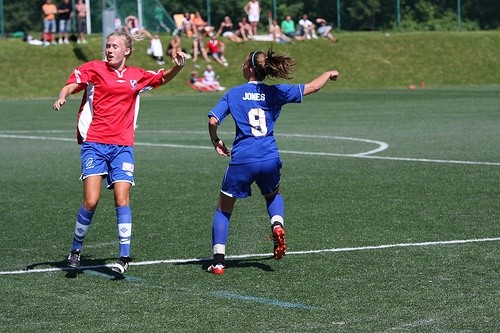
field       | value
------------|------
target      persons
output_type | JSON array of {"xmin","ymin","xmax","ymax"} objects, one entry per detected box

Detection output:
[
  {"xmin": 316, "ymin": 18, "xmax": 338, "ymax": 44},
  {"xmin": 57, "ymin": 0, "xmax": 73, "ymax": 45},
  {"xmin": 280, "ymin": 15, "xmax": 307, "ymax": 41},
  {"xmin": 190, "ymin": 64, "xmax": 227, "ymax": 93},
  {"xmin": 166, "ymin": 34, "xmax": 187, "ymax": 64},
  {"xmin": 244, "ymin": 0, "xmax": 260, "ymax": 36},
  {"xmin": 146, "ymin": 34, "xmax": 165, "ymax": 61},
  {"xmin": 206, "ymin": 36, "xmax": 229, "ymax": 67},
  {"xmin": 108, "ymin": 5, "xmax": 259, "ymax": 47},
  {"xmin": 296, "ymin": 15, "xmax": 318, "ymax": 40},
  {"xmin": 190, "ymin": 34, "xmax": 208, "ymax": 62},
  {"xmin": 269, "ymin": 20, "xmax": 291, "ymax": 44},
  {"xmin": 203, "ymin": 50, "xmax": 340, "ymax": 276},
  {"xmin": 75, "ymin": 0, "xmax": 88, "ymax": 45},
  {"xmin": 41, "ymin": 0, "xmax": 58, "ymax": 46},
  {"xmin": 52, "ymin": 31, "xmax": 187, "ymax": 274}
]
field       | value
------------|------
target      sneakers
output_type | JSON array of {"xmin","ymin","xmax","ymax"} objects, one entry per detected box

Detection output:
[
  {"xmin": 66, "ymin": 249, "xmax": 81, "ymax": 268},
  {"xmin": 207, "ymin": 263, "xmax": 225, "ymax": 275},
  {"xmin": 272, "ymin": 226, "xmax": 287, "ymax": 260},
  {"xmin": 112, "ymin": 257, "xmax": 133, "ymax": 274}
]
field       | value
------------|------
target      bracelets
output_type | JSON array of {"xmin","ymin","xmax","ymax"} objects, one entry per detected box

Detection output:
[{"xmin": 212, "ymin": 137, "xmax": 219, "ymax": 144}]
